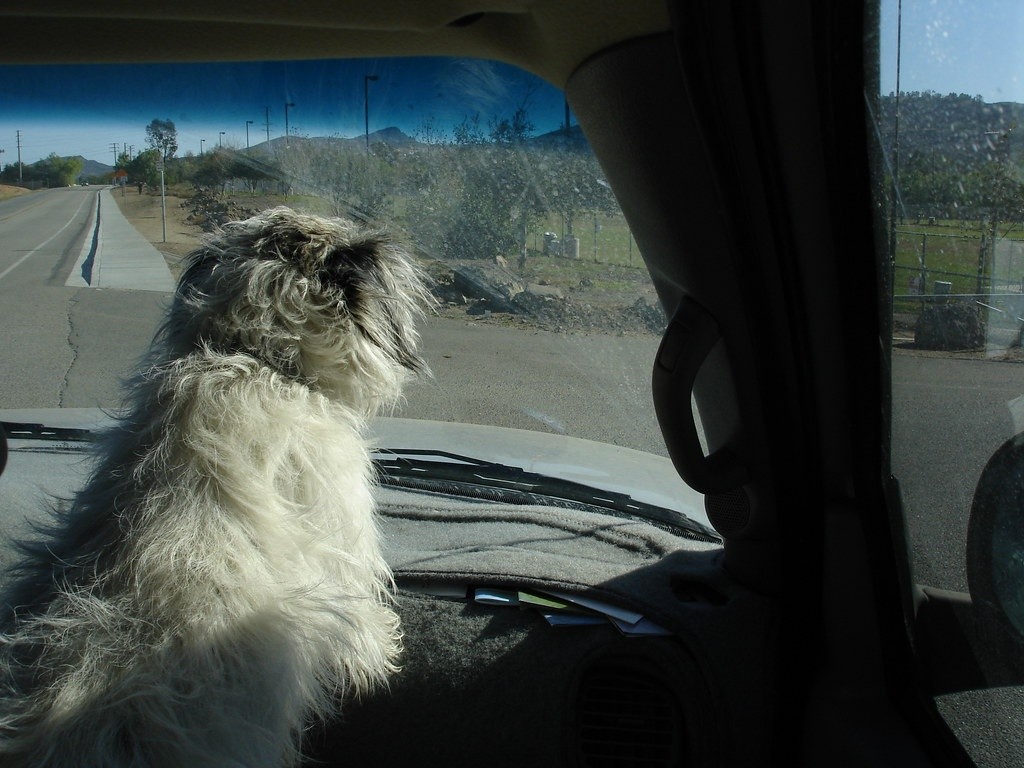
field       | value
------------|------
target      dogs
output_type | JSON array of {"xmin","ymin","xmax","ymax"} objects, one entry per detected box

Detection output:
[{"xmin": 1, "ymin": 206, "xmax": 444, "ymax": 768}]
